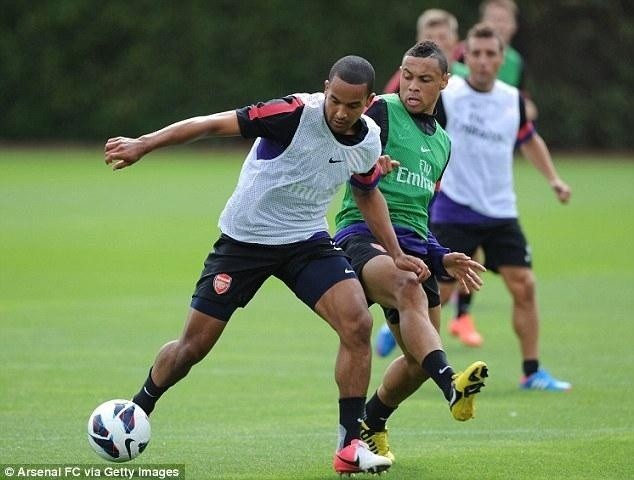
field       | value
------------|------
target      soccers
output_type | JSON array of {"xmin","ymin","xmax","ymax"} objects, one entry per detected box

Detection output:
[{"xmin": 87, "ymin": 398, "xmax": 153, "ymax": 463}]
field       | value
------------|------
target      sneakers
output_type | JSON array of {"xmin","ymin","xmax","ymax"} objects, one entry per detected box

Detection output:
[
  {"xmin": 519, "ymin": 368, "xmax": 572, "ymax": 393},
  {"xmin": 333, "ymin": 438, "xmax": 392, "ymax": 474},
  {"xmin": 376, "ymin": 322, "xmax": 397, "ymax": 357},
  {"xmin": 448, "ymin": 360, "xmax": 488, "ymax": 420},
  {"xmin": 361, "ymin": 420, "xmax": 396, "ymax": 464},
  {"xmin": 449, "ymin": 314, "xmax": 484, "ymax": 346}
]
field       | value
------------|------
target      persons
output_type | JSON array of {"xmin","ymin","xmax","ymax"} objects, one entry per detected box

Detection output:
[
  {"xmin": 380, "ymin": 5, "xmax": 467, "ymax": 128},
  {"xmin": 374, "ymin": 20, "xmax": 582, "ymax": 391},
  {"xmin": 333, "ymin": 42, "xmax": 489, "ymax": 462},
  {"xmin": 101, "ymin": 57, "xmax": 439, "ymax": 476},
  {"xmin": 455, "ymin": 0, "xmax": 540, "ymax": 120}
]
